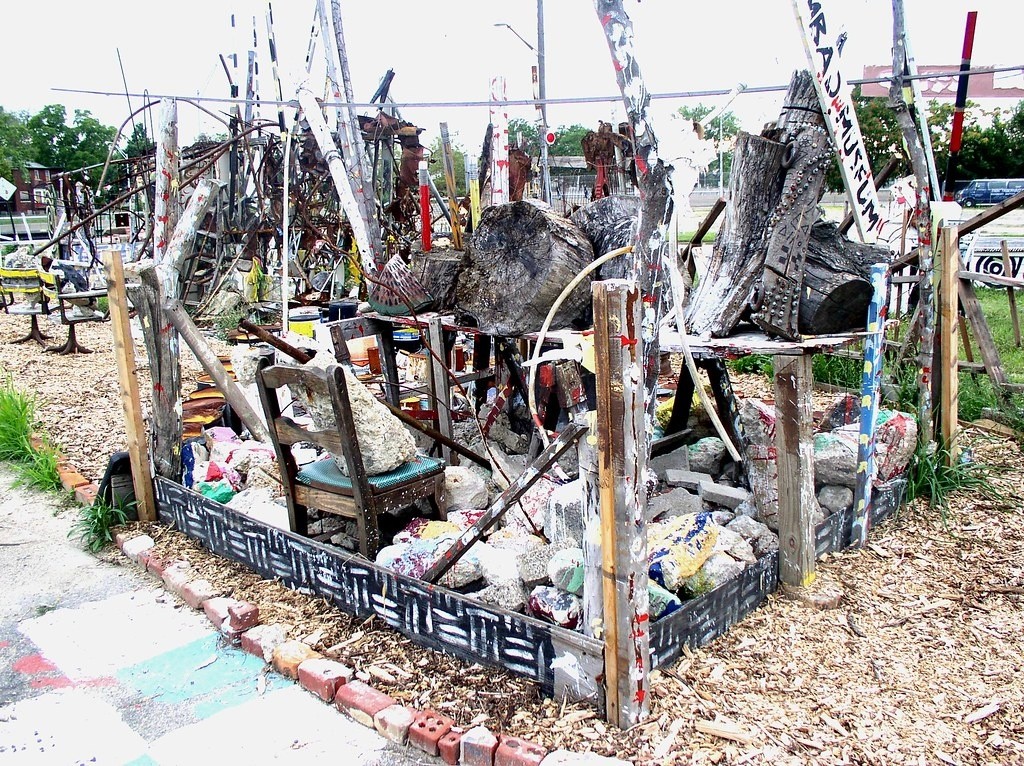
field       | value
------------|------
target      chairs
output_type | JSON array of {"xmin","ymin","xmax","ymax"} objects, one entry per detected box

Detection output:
[
  {"xmin": 36, "ymin": 271, "xmax": 110, "ymax": 355},
  {"xmin": 252, "ymin": 355, "xmax": 449, "ymax": 563},
  {"xmin": 0, "ymin": 267, "xmax": 56, "ymax": 349}
]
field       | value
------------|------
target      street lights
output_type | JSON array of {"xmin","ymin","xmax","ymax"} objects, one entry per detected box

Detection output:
[{"xmin": 493, "ymin": 22, "xmax": 551, "ymax": 205}]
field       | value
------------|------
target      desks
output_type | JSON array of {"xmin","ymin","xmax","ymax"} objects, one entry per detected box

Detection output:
[{"xmin": 360, "ymin": 311, "xmax": 899, "ymax": 588}]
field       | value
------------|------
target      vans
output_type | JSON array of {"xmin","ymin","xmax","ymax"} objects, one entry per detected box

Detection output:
[{"xmin": 956, "ymin": 178, "xmax": 1024, "ymax": 208}]
